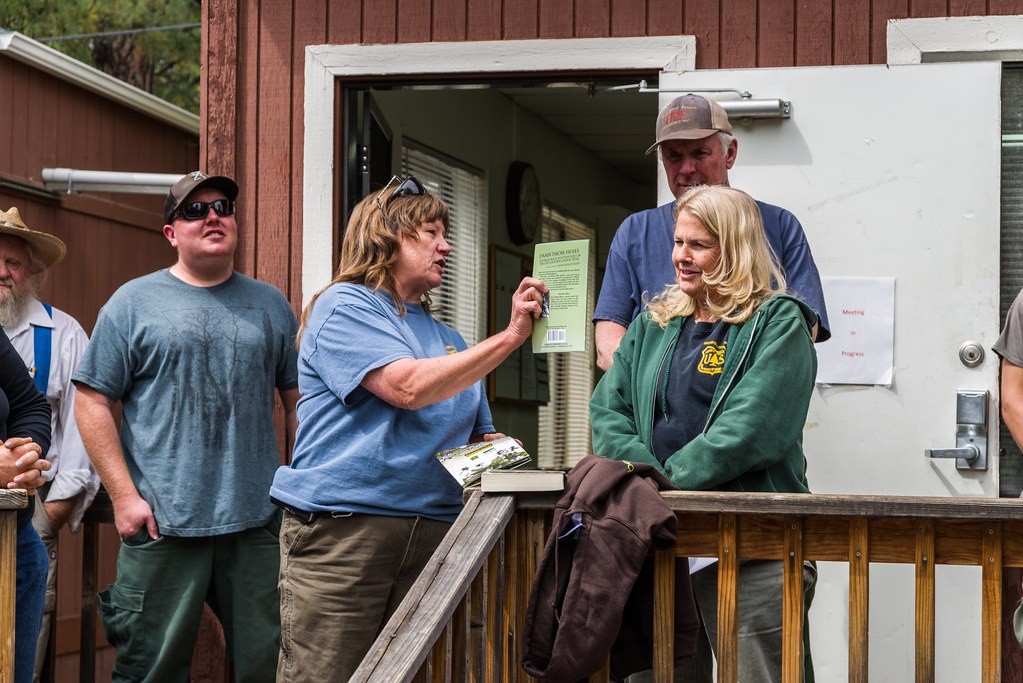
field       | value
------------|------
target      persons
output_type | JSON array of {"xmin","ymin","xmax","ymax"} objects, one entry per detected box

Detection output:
[
  {"xmin": 264, "ymin": 172, "xmax": 550, "ymax": 681},
  {"xmin": 0, "ymin": 203, "xmax": 107, "ymax": 683},
  {"xmin": 592, "ymin": 94, "xmax": 833, "ymax": 379},
  {"xmin": 991, "ymin": 287, "xmax": 1022, "ymax": 502},
  {"xmin": 69, "ymin": 169, "xmax": 305, "ymax": 683},
  {"xmin": 585, "ymin": 184, "xmax": 824, "ymax": 683}
]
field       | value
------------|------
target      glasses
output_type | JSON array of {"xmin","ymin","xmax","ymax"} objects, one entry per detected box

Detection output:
[
  {"xmin": 170, "ymin": 198, "xmax": 236, "ymax": 225},
  {"xmin": 386, "ymin": 177, "xmax": 429, "ymax": 205},
  {"xmin": 374, "ymin": 174, "xmax": 404, "ymax": 203}
]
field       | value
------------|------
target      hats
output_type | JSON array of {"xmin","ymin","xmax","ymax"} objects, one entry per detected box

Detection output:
[
  {"xmin": 164, "ymin": 171, "xmax": 239, "ymax": 223},
  {"xmin": 645, "ymin": 94, "xmax": 733, "ymax": 157},
  {"xmin": 0, "ymin": 207, "xmax": 67, "ymax": 277}
]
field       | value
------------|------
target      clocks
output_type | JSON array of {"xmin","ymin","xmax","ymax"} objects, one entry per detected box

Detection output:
[{"xmin": 504, "ymin": 160, "xmax": 541, "ymax": 245}]
